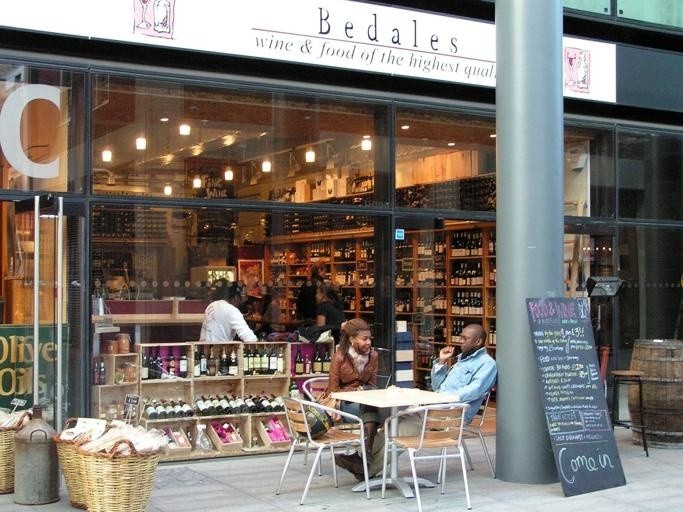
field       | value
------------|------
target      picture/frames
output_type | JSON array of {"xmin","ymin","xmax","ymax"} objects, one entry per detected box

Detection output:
[{"xmin": 237, "ymin": 259, "xmax": 264, "ymax": 296}]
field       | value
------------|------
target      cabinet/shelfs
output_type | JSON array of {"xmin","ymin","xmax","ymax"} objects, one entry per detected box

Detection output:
[
  {"xmin": 267, "ymin": 225, "xmax": 385, "ymax": 346},
  {"xmin": 89, "ymin": 352, "xmax": 140, "ymax": 431},
  {"xmin": 134, "ymin": 342, "xmax": 335, "ymax": 463},
  {"xmin": 409, "ymin": 144, "xmax": 487, "ymax": 186},
  {"xmin": 392, "ymin": 224, "xmax": 497, "ymax": 405}
]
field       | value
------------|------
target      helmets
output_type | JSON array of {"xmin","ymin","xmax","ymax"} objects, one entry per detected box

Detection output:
[{"xmin": 297, "ymin": 401, "xmax": 333, "ymax": 440}]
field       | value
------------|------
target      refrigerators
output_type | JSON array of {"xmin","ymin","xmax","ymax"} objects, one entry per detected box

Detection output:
[{"xmin": 190, "ymin": 266, "xmax": 236, "ymax": 285}]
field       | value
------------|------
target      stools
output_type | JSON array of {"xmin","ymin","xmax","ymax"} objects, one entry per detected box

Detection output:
[{"xmin": 608, "ymin": 368, "xmax": 648, "ymax": 458}]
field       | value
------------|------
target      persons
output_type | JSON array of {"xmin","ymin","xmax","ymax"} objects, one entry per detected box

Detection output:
[
  {"xmin": 329, "ymin": 318, "xmax": 378, "ymax": 483},
  {"xmin": 197, "ymin": 260, "xmax": 346, "ymax": 345},
  {"xmin": 334, "ymin": 324, "xmax": 498, "ymax": 482}
]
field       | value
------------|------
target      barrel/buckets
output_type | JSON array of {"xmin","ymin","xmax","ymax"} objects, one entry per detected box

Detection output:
[
  {"xmin": 625, "ymin": 337, "xmax": 682, "ymax": 452},
  {"xmin": 11, "ymin": 429, "xmax": 61, "ymax": 506}
]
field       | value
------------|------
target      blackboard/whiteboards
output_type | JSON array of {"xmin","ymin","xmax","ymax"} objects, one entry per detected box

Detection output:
[{"xmin": 525, "ymin": 297, "xmax": 627, "ymax": 497}]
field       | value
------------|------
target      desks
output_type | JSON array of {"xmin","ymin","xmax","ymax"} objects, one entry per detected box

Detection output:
[
  {"xmin": 100, "ymin": 312, "xmax": 211, "ymax": 341},
  {"xmin": 243, "ymin": 317, "xmax": 306, "ymax": 341}
]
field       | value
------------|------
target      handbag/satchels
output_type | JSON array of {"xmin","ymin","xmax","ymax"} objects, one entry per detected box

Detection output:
[{"xmin": 316, "ymin": 387, "xmax": 341, "ymax": 423}]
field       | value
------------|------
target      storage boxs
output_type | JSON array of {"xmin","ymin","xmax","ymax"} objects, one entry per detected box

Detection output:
[{"xmin": 290, "ymin": 162, "xmax": 360, "ymax": 203}]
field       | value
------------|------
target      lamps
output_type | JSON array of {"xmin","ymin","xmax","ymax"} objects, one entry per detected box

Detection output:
[
  {"xmin": 585, "ymin": 276, "xmax": 625, "ymax": 299},
  {"xmin": 101, "ymin": 121, "xmax": 373, "ymax": 196}
]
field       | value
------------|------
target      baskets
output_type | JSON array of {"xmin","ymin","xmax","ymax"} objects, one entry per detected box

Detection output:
[
  {"xmin": 74, "ymin": 437, "xmax": 161, "ymax": 512},
  {"xmin": 0, "ymin": 412, "xmax": 32, "ymax": 494},
  {"xmin": 52, "ymin": 417, "xmax": 87, "ymax": 511}
]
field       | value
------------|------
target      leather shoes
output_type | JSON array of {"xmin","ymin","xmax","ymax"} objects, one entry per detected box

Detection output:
[
  {"xmin": 353, "ymin": 473, "xmax": 372, "ymax": 482},
  {"xmin": 334, "ymin": 452, "xmax": 363, "ymax": 474}
]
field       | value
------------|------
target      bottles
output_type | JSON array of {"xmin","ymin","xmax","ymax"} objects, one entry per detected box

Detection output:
[
  {"xmin": 142, "ymin": 392, "xmax": 287, "ymax": 420},
  {"xmin": 210, "ymin": 271, "xmax": 228, "ymax": 282},
  {"xmin": 342, "ymin": 294, "xmax": 411, "ymax": 314},
  {"xmin": 323, "ymin": 264, "xmax": 410, "ymax": 288},
  {"xmin": 101, "ymin": 400, "xmax": 136, "ymax": 422},
  {"xmin": 142, "ymin": 345, "xmax": 284, "ymax": 380},
  {"xmin": 98, "ymin": 358, "xmax": 105, "ymax": 384},
  {"xmin": 369, "ymin": 323, "xmax": 373, "ymax": 337},
  {"xmin": 322, "ymin": 343, "xmax": 331, "ymax": 372},
  {"xmin": 414, "ymin": 230, "xmax": 495, "ymax": 368},
  {"xmin": 91, "ymin": 252, "xmax": 134, "ymax": 269},
  {"xmin": 288, "ymin": 380, "xmax": 299, "ymax": 399},
  {"xmin": 92, "ymin": 358, "xmax": 98, "ymax": 385},
  {"xmin": 91, "ymin": 202, "xmax": 166, "ymax": 239},
  {"xmin": 167, "ymin": 415, "xmax": 288, "ymax": 453},
  {"xmin": 309, "ymin": 239, "xmax": 414, "ymax": 261},
  {"xmin": 295, "ymin": 345, "xmax": 311, "ymax": 374},
  {"xmin": 312, "ymin": 343, "xmax": 322, "ymax": 372},
  {"xmin": 113, "ymin": 362, "xmax": 136, "ymax": 384}
]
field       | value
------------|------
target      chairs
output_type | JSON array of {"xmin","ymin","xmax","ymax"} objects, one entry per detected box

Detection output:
[{"xmin": 275, "ymin": 369, "xmax": 498, "ymax": 512}]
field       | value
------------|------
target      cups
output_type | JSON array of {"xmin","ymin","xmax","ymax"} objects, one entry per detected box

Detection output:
[
  {"xmin": 118, "ymin": 333, "xmax": 130, "ymax": 353},
  {"xmin": 105, "ymin": 339, "xmax": 118, "ymax": 354},
  {"xmin": 93, "ymin": 297, "xmax": 103, "ymax": 322}
]
